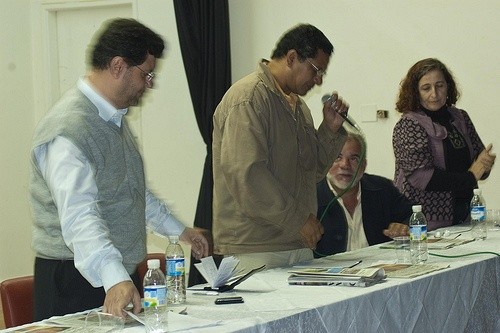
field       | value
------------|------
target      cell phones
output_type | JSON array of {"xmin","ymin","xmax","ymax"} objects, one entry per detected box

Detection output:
[{"xmin": 215, "ymin": 297, "xmax": 244, "ymax": 305}]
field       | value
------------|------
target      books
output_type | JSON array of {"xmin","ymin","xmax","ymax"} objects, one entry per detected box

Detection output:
[{"xmin": 193, "ymin": 255, "xmax": 267, "ymax": 293}]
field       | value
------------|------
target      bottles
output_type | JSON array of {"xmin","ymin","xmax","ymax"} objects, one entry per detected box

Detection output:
[
  {"xmin": 143, "ymin": 259, "xmax": 168, "ymax": 333},
  {"xmin": 409, "ymin": 205, "xmax": 428, "ymax": 265},
  {"xmin": 470, "ymin": 189, "xmax": 488, "ymax": 241},
  {"xmin": 165, "ymin": 235, "xmax": 186, "ymax": 306}
]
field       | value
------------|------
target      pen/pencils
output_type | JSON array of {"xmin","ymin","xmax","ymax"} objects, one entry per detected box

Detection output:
[{"xmin": 192, "ymin": 292, "xmax": 219, "ymax": 296}]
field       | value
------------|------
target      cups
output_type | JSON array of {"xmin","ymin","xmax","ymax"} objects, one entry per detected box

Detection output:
[
  {"xmin": 85, "ymin": 310, "xmax": 124, "ymax": 333},
  {"xmin": 393, "ymin": 237, "xmax": 410, "ymax": 263},
  {"xmin": 487, "ymin": 208, "xmax": 500, "ymax": 230}
]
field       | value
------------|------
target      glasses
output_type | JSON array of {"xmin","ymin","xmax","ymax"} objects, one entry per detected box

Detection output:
[
  {"xmin": 133, "ymin": 65, "xmax": 155, "ymax": 89},
  {"xmin": 305, "ymin": 56, "xmax": 326, "ymax": 80}
]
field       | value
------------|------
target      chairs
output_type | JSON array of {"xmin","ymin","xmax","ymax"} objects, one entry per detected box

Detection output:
[
  {"xmin": 0, "ymin": 275, "xmax": 34, "ymax": 328},
  {"xmin": 137, "ymin": 253, "xmax": 165, "ymax": 298}
]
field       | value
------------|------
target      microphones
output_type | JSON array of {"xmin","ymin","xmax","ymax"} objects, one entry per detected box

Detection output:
[{"xmin": 321, "ymin": 94, "xmax": 360, "ymax": 132}]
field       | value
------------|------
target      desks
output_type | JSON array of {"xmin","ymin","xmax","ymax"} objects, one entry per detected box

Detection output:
[{"xmin": 0, "ymin": 222, "xmax": 500, "ymax": 333}]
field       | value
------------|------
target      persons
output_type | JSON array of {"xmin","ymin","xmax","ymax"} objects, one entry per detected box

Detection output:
[
  {"xmin": 392, "ymin": 58, "xmax": 496, "ymax": 232},
  {"xmin": 30, "ymin": 17, "xmax": 209, "ymax": 323},
  {"xmin": 311, "ymin": 133, "xmax": 419, "ymax": 259},
  {"xmin": 212, "ymin": 23, "xmax": 349, "ymax": 273}
]
